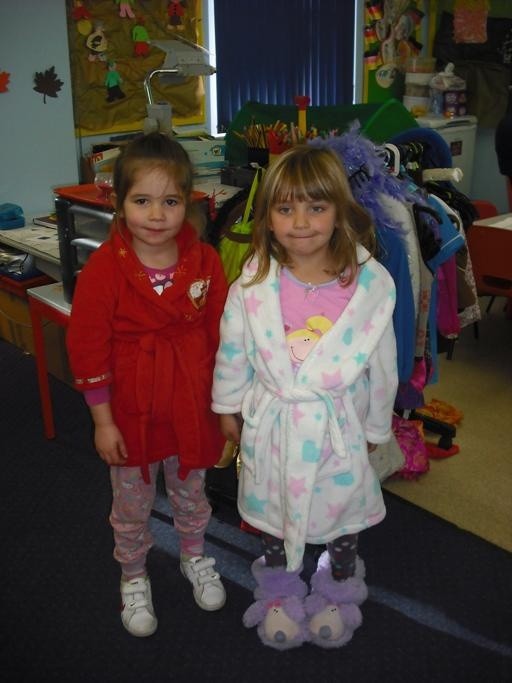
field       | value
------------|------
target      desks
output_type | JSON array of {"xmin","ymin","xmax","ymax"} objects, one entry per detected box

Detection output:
[
  {"xmin": 0, "ymin": 226, "xmax": 62, "ymax": 282},
  {"xmin": 26, "ymin": 281, "xmax": 73, "ymax": 440},
  {"xmin": 472, "ymin": 212, "xmax": 512, "ymax": 231}
]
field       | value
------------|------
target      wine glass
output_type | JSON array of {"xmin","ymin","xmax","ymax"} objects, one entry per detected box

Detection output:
[{"xmin": 94, "ymin": 171, "xmax": 114, "ymax": 202}]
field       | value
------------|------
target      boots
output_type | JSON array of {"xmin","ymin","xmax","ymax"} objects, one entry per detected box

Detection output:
[{"xmin": 242, "ymin": 550, "xmax": 368, "ymax": 651}]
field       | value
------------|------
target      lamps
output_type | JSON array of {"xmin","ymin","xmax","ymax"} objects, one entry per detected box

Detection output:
[{"xmin": 144, "ymin": 62, "xmax": 217, "ymax": 136}]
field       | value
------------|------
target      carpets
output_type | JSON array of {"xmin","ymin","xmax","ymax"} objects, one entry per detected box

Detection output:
[{"xmin": 0, "ymin": 337, "xmax": 512, "ymax": 682}]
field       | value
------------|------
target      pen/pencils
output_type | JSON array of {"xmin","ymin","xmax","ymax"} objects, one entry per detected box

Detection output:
[{"xmin": 230, "ymin": 115, "xmax": 340, "ymax": 150}]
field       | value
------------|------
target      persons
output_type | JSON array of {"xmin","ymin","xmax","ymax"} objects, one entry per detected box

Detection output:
[
  {"xmin": 65, "ymin": 131, "xmax": 229, "ymax": 639},
  {"xmin": 209, "ymin": 143, "xmax": 398, "ymax": 650}
]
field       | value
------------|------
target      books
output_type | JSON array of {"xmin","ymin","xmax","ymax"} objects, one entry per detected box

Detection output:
[
  {"xmin": 34, "ymin": 215, "xmax": 58, "ymax": 229},
  {"xmin": 83, "ymin": 128, "xmax": 246, "ymax": 208}
]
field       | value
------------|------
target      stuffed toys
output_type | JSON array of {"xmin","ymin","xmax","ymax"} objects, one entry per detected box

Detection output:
[
  {"xmin": 72, "ymin": 0, "xmax": 92, "ymax": 19},
  {"xmin": 86, "ymin": 18, "xmax": 109, "ymax": 62},
  {"xmin": 104, "ymin": 59, "xmax": 126, "ymax": 102},
  {"xmin": 114, "ymin": 0, "xmax": 137, "ymax": 19},
  {"xmin": 132, "ymin": 16, "xmax": 151, "ymax": 57},
  {"xmin": 166, "ymin": 0, "xmax": 187, "ymax": 31}
]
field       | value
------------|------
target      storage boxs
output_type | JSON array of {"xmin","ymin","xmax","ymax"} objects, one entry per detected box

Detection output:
[{"xmin": 0, "ymin": 289, "xmax": 62, "ymax": 382}]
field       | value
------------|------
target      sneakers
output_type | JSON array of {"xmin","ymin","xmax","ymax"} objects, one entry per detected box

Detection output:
[
  {"xmin": 180, "ymin": 551, "xmax": 227, "ymax": 611},
  {"xmin": 120, "ymin": 572, "xmax": 158, "ymax": 637}
]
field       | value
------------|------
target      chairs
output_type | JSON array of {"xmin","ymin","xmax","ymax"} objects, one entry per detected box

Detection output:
[
  {"xmin": 447, "ymin": 225, "xmax": 512, "ymax": 361},
  {"xmin": 469, "ymin": 200, "xmax": 498, "ymax": 219}
]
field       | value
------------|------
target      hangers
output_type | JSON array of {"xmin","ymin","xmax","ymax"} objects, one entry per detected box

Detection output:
[{"xmin": 375, "ymin": 140, "xmax": 460, "ymax": 251}]
